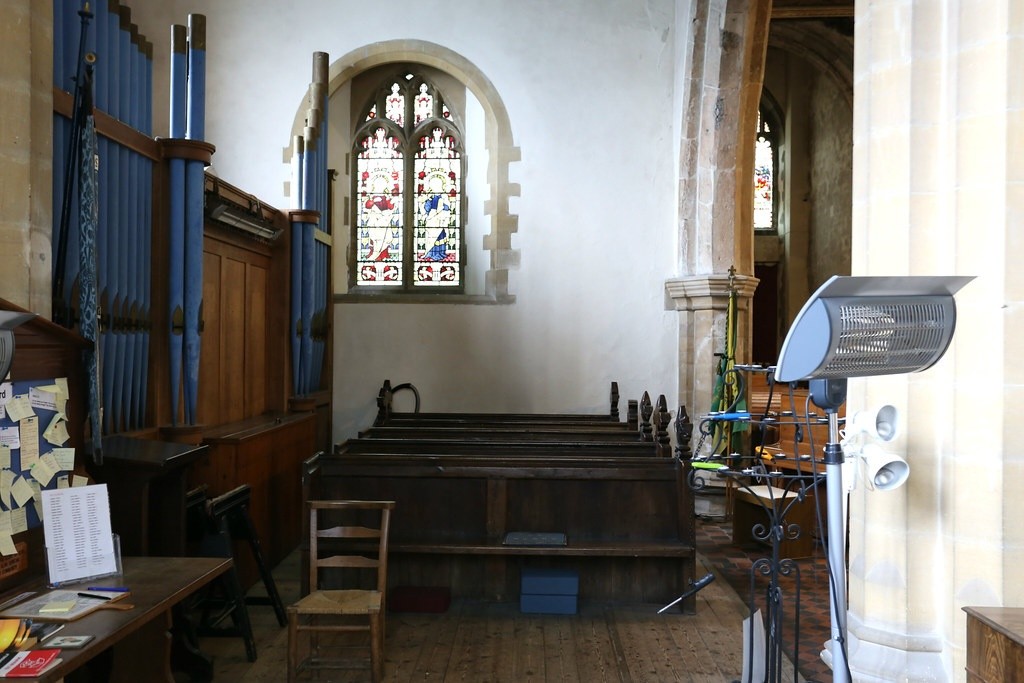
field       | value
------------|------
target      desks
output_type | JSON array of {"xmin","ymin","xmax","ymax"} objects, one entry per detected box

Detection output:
[
  {"xmin": 84, "ymin": 435, "xmax": 215, "ymax": 683},
  {"xmin": 0, "ymin": 555, "xmax": 234, "ymax": 683}
]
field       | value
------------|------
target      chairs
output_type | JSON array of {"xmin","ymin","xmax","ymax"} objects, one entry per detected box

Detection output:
[{"xmin": 283, "ymin": 498, "xmax": 395, "ymax": 683}]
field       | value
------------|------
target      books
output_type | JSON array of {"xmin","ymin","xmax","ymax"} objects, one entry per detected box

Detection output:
[{"xmin": 0, "ymin": 619, "xmax": 65, "ymax": 677}]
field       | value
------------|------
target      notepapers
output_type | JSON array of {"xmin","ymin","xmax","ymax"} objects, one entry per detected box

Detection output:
[{"xmin": 38, "ymin": 601, "xmax": 76, "ymax": 612}]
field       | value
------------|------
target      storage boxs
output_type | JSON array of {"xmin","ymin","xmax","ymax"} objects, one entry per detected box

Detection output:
[
  {"xmin": 520, "ymin": 594, "xmax": 578, "ymax": 615},
  {"xmin": 519, "ymin": 568, "xmax": 580, "ymax": 596},
  {"xmin": 397, "ymin": 586, "xmax": 452, "ymax": 615}
]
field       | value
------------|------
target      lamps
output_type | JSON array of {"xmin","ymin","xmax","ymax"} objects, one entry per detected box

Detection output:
[
  {"xmin": 203, "ymin": 178, "xmax": 284, "ymax": 243},
  {"xmin": 835, "ymin": 405, "xmax": 910, "ymax": 611},
  {"xmin": 772, "ymin": 271, "xmax": 977, "ymax": 683}
]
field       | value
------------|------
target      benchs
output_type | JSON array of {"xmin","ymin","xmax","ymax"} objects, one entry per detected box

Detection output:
[
  {"xmin": 756, "ymin": 391, "xmax": 847, "ymax": 539},
  {"xmin": 300, "ymin": 378, "xmax": 696, "ymax": 617},
  {"xmin": 731, "ymin": 484, "xmax": 815, "ymax": 561}
]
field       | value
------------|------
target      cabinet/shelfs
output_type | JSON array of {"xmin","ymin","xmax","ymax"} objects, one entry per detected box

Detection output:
[
  {"xmin": 962, "ymin": 605, "xmax": 1024, "ymax": 683},
  {"xmin": 189, "ymin": 410, "xmax": 316, "ymax": 598}
]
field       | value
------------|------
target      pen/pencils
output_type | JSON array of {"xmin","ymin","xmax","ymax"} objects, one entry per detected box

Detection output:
[
  {"xmin": 78, "ymin": 593, "xmax": 111, "ymax": 600},
  {"xmin": 88, "ymin": 587, "xmax": 130, "ymax": 592}
]
